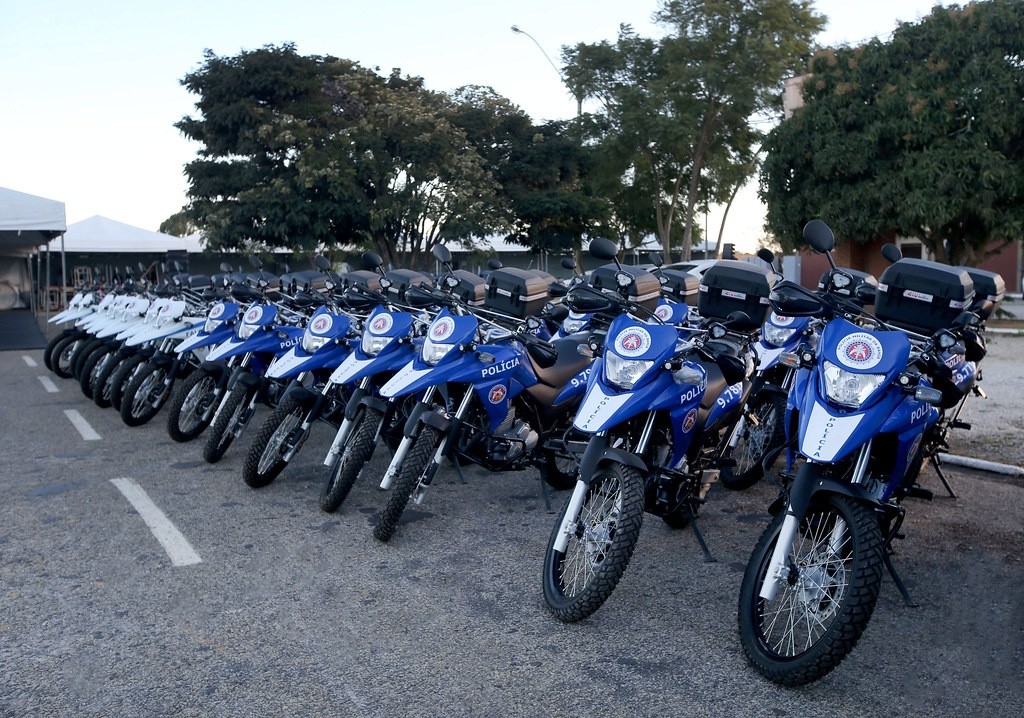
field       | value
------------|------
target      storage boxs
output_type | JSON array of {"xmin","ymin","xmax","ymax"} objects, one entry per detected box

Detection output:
[{"xmin": 171, "ymin": 256, "xmax": 1006, "ymax": 341}]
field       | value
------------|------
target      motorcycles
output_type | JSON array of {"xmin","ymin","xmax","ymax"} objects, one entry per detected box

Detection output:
[
  {"xmin": 540, "ymin": 236, "xmax": 813, "ymax": 624},
  {"xmin": 315, "ymin": 266, "xmax": 547, "ymax": 514},
  {"xmin": 43, "ymin": 260, "xmax": 383, "ymax": 491},
  {"xmin": 736, "ymin": 258, "xmax": 1008, "ymax": 689},
  {"xmin": 376, "ymin": 265, "xmax": 663, "ymax": 543}
]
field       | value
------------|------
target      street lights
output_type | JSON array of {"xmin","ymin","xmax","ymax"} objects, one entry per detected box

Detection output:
[{"xmin": 509, "ymin": 25, "xmax": 585, "ymax": 119}]
